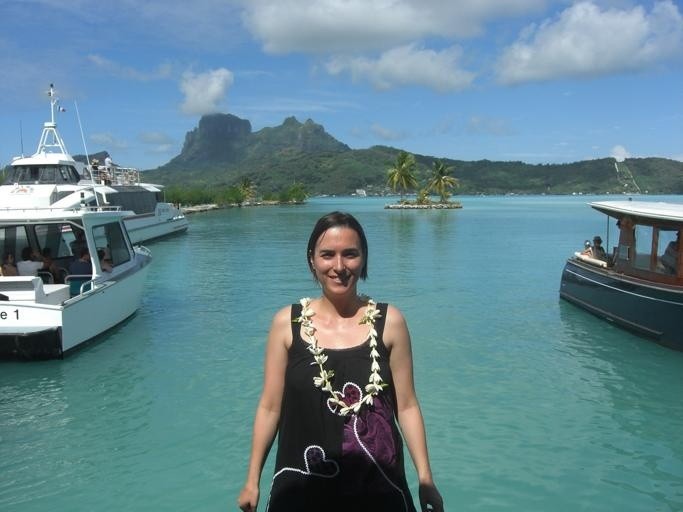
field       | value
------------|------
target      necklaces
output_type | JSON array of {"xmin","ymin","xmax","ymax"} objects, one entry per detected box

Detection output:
[{"xmin": 297, "ymin": 293, "xmax": 384, "ymax": 419}]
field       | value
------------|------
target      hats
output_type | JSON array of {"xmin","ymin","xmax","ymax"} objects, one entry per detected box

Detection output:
[
  {"xmin": 593, "ymin": 236, "xmax": 603, "ymax": 242},
  {"xmin": 92, "ymin": 159, "xmax": 99, "ymax": 164}
]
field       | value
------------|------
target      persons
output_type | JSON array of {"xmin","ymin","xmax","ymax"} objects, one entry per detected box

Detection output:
[
  {"xmin": 15, "ymin": 244, "xmax": 50, "ymax": 276},
  {"xmin": 104, "ymin": 152, "xmax": 114, "ymax": 170},
  {"xmin": 96, "ymin": 249, "xmax": 113, "ymax": 273},
  {"xmin": 234, "ymin": 208, "xmax": 443, "ymax": 511},
  {"xmin": 66, "ymin": 247, "xmax": 93, "ymax": 295},
  {"xmin": 0, "ymin": 249, "xmax": 18, "ymax": 275},
  {"xmin": 581, "ymin": 235, "xmax": 611, "ymax": 267},
  {"xmin": 40, "ymin": 246, "xmax": 62, "ymax": 280},
  {"xmin": 661, "ymin": 232, "xmax": 678, "ymax": 274},
  {"xmin": 90, "ymin": 157, "xmax": 111, "ymax": 186}
]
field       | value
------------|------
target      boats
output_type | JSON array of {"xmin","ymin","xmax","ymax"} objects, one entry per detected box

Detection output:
[
  {"xmin": 560, "ymin": 202, "xmax": 683, "ymax": 353},
  {"xmin": 0, "ymin": 205, "xmax": 154, "ymax": 359},
  {"xmin": 0, "ymin": 83, "xmax": 189, "ymax": 260}
]
